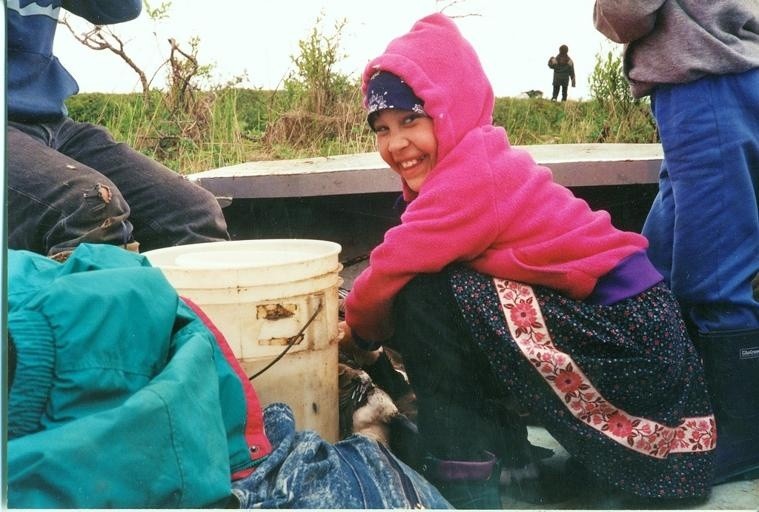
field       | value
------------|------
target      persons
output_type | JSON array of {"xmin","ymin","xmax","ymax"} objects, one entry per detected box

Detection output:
[
  {"xmin": 594, "ymin": 0, "xmax": 759, "ymax": 485},
  {"xmin": 8, "ymin": 0, "xmax": 230, "ymax": 262},
  {"xmin": 344, "ymin": 13, "xmax": 719, "ymax": 509},
  {"xmin": 548, "ymin": 45, "xmax": 577, "ymax": 101}
]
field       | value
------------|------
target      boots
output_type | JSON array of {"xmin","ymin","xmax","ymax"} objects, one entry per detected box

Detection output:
[
  {"xmin": 689, "ymin": 331, "xmax": 759, "ymax": 487},
  {"xmin": 413, "ymin": 449, "xmax": 502, "ymax": 509}
]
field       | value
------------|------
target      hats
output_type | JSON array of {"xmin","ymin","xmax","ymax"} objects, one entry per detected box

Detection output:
[{"xmin": 367, "ymin": 71, "xmax": 429, "ymax": 130}]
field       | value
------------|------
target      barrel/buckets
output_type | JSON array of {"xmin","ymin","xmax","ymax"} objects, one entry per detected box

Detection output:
[{"xmin": 146, "ymin": 238, "xmax": 342, "ymax": 443}]
